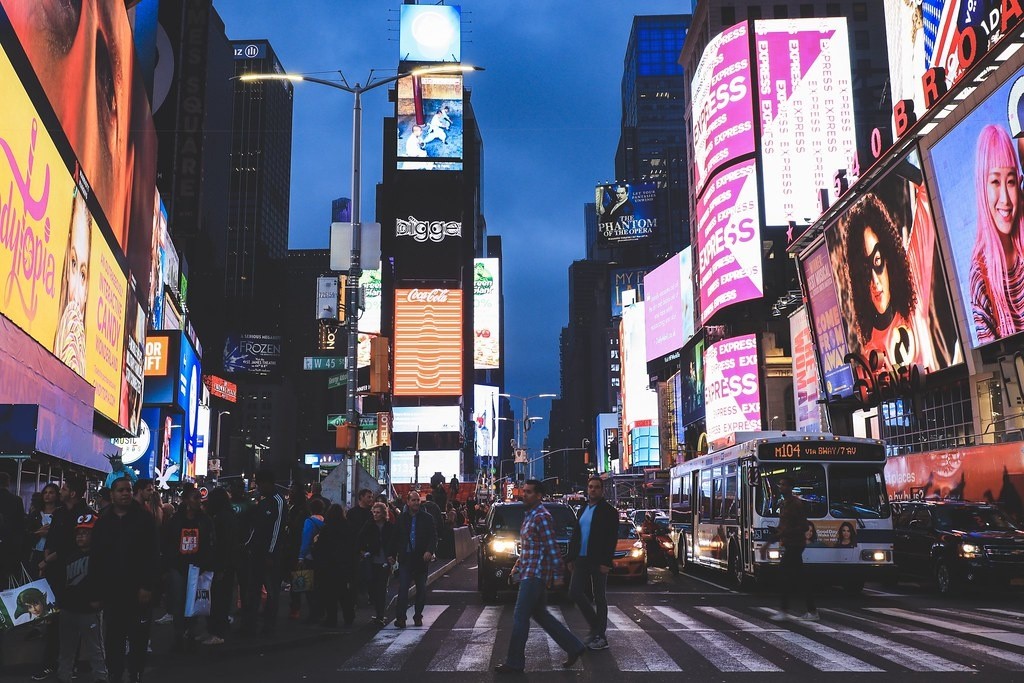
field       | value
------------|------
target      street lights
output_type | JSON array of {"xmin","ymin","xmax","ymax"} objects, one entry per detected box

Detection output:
[
  {"xmin": 492, "ymin": 393, "xmax": 557, "ymax": 480},
  {"xmin": 216, "ymin": 410, "xmax": 230, "ymax": 457},
  {"xmin": 240, "ymin": 63, "xmax": 484, "ymax": 466}
]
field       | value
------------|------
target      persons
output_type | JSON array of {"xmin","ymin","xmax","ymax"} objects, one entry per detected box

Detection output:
[
  {"xmin": 642, "ymin": 511, "xmax": 671, "ymax": 562},
  {"xmin": 53, "ymin": 191, "xmax": 92, "ymax": 370},
  {"xmin": 495, "ymin": 480, "xmax": 586, "ymax": 672},
  {"xmin": 247, "ymin": 469, "xmax": 287, "ymax": 627},
  {"xmin": 0, "ymin": 472, "xmax": 240, "ymax": 683},
  {"xmin": 969, "ymin": 124, "xmax": 1024, "ymax": 346},
  {"xmin": 421, "ymin": 493, "xmax": 441, "ymax": 561},
  {"xmin": 347, "ymin": 489, "xmax": 373, "ymax": 529},
  {"xmin": 838, "ymin": 522, "xmax": 856, "ymax": 548},
  {"xmin": 405, "ymin": 106, "xmax": 454, "ymax": 158},
  {"xmin": 759, "ymin": 476, "xmax": 820, "ymax": 622},
  {"xmin": 602, "ymin": 185, "xmax": 634, "ymax": 246},
  {"xmin": 288, "ymin": 481, "xmax": 347, "ymax": 621},
  {"xmin": 356, "ymin": 502, "xmax": 397, "ymax": 626},
  {"xmin": 805, "ymin": 522, "xmax": 818, "ymax": 546},
  {"xmin": 847, "ymin": 194, "xmax": 935, "ymax": 401},
  {"xmin": 388, "ymin": 491, "xmax": 438, "ymax": 627},
  {"xmin": 14, "ymin": 587, "xmax": 53, "ymax": 620},
  {"xmin": 449, "ymin": 475, "xmax": 459, "ymax": 500},
  {"xmin": 575, "ymin": 477, "xmax": 618, "ymax": 649},
  {"xmin": 446, "ymin": 503, "xmax": 457, "ymax": 554}
]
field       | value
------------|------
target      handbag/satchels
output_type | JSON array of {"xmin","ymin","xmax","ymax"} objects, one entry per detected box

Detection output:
[
  {"xmin": 184, "ymin": 563, "xmax": 214, "ymax": 616},
  {"xmin": 291, "ymin": 562, "xmax": 315, "ymax": 591},
  {"xmin": 0, "ymin": 561, "xmax": 60, "ymax": 634}
]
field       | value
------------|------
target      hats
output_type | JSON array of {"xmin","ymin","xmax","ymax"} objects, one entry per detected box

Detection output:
[{"xmin": 72, "ymin": 513, "xmax": 98, "ymax": 529}]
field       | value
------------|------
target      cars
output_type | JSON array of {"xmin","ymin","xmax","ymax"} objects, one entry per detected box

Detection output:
[
  {"xmin": 542, "ymin": 492, "xmax": 672, "ymax": 525},
  {"xmin": 605, "ymin": 520, "xmax": 652, "ymax": 587}
]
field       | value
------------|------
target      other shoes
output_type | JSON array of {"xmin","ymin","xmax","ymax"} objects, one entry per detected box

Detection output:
[
  {"xmin": 797, "ymin": 610, "xmax": 820, "ymax": 620},
  {"xmin": 394, "ymin": 620, "xmax": 406, "ymax": 627},
  {"xmin": 32, "ymin": 662, "xmax": 60, "ymax": 680},
  {"xmin": 414, "ymin": 618, "xmax": 422, "ymax": 626},
  {"xmin": 771, "ymin": 611, "xmax": 787, "ymax": 621},
  {"xmin": 70, "ymin": 605, "xmax": 388, "ymax": 678},
  {"xmin": 583, "ymin": 634, "xmax": 608, "ymax": 650},
  {"xmin": 25, "ymin": 628, "xmax": 43, "ymax": 640},
  {"xmin": 562, "ymin": 647, "xmax": 586, "ymax": 667},
  {"xmin": 495, "ymin": 664, "xmax": 524, "ymax": 672}
]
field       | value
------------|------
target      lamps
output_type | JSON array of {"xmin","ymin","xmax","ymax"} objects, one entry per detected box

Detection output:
[
  {"xmin": 804, "ymin": 218, "xmax": 815, "ymax": 223},
  {"xmin": 772, "ymin": 290, "xmax": 802, "ymax": 316}
]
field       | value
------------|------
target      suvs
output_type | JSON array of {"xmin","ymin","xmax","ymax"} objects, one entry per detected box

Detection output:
[
  {"xmin": 476, "ymin": 500, "xmax": 587, "ymax": 607},
  {"xmin": 889, "ymin": 498, "xmax": 1024, "ymax": 608}
]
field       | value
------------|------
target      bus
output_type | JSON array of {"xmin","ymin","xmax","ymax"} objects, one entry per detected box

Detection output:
[{"xmin": 671, "ymin": 430, "xmax": 895, "ymax": 594}]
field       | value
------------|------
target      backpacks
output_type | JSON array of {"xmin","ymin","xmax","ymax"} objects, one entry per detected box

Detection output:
[{"xmin": 309, "ymin": 516, "xmax": 321, "ymax": 558}]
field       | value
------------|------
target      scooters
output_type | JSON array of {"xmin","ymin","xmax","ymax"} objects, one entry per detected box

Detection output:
[{"xmin": 642, "ymin": 526, "xmax": 681, "ymax": 577}]
